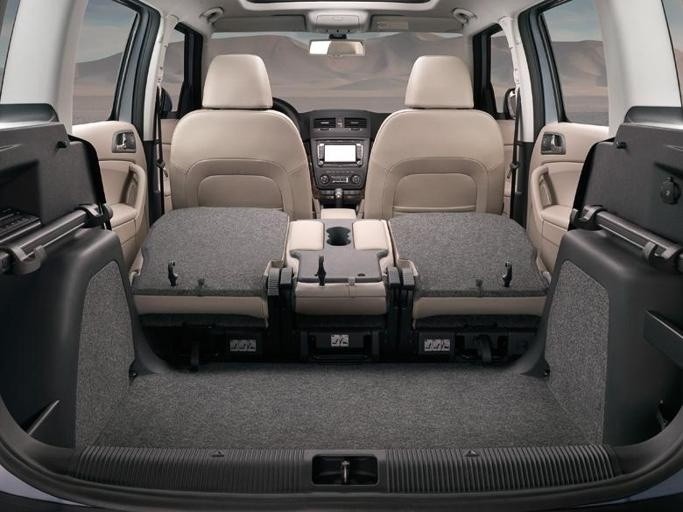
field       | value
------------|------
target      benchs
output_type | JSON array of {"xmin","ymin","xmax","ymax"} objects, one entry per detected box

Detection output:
[{"xmin": 128, "ymin": 206, "xmax": 547, "ymax": 363}]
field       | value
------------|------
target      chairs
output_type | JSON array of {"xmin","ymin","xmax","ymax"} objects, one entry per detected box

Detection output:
[
  {"xmin": 364, "ymin": 54, "xmax": 504, "ymax": 219},
  {"xmin": 170, "ymin": 53, "xmax": 313, "ymax": 219}
]
